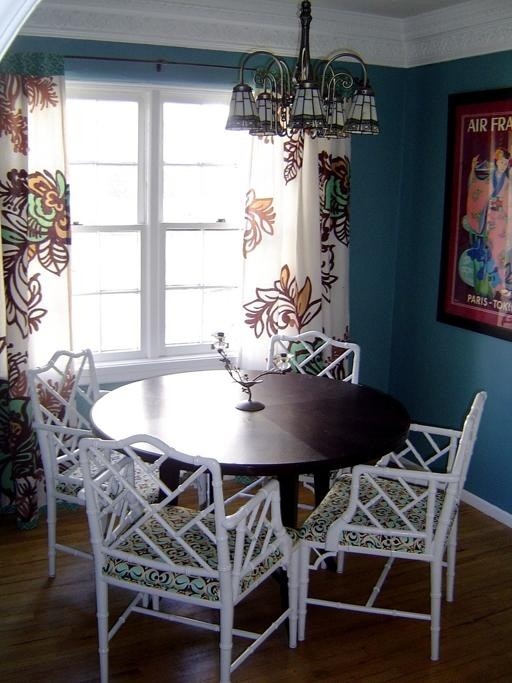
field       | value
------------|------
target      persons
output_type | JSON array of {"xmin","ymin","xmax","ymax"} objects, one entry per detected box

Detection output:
[{"xmin": 466, "ymin": 147, "xmax": 512, "ymax": 296}]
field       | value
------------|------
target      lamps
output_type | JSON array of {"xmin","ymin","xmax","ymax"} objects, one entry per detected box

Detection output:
[
  {"xmin": 226, "ymin": 0, "xmax": 380, "ymax": 136},
  {"xmin": 207, "ymin": 329, "xmax": 295, "ymax": 414}
]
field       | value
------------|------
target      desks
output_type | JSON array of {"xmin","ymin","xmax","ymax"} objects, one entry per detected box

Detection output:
[{"xmin": 88, "ymin": 371, "xmax": 412, "ymax": 626}]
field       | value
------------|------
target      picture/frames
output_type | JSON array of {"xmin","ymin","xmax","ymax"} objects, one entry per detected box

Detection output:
[{"xmin": 434, "ymin": 83, "xmax": 512, "ymax": 339}]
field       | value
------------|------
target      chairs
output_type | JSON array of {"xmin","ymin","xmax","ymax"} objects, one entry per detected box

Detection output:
[
  {"xmin": 294, "ymin": 387, "xmax": 489, "ymax": 662},
  {"xmin": 220, "ymin": 327, "xmax": 362, "ymax": 581},
  {"xmin": 26, "ymin": 347, "xmax": 175, "ymax": 613},
  {"xmin": 78, "ymin": 431, "xmax": 302, "ymax": 683}
]
